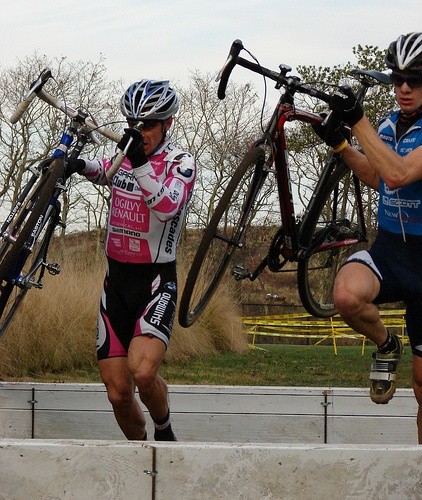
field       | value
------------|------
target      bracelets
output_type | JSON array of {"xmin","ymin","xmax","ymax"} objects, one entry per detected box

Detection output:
[{"xmin": 332, "ymin": 139, "xmax": 348, "ymax": 153}]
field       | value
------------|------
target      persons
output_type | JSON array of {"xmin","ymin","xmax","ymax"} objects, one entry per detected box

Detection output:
[
  {"xmin": 311, "ymin": 32, "xmax": 422, "ymax": 445},
  {"xmin": 37, "ymin": 79, "xmax": 195, "ymax": 442}
]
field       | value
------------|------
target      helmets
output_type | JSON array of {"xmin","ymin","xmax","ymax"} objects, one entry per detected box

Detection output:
[
  {"xmin": 384, "ymin": 32, "xmax": 422, "ymax": 74},
  {"xmin": 120, "ymin": 79, "xmax": 179, "ymax": 120}
]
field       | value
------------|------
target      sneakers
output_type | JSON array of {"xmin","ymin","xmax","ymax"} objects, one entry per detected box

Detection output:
[{"xmin": 369, "ymin": 331, "xmax": 404, "ymax": 403}]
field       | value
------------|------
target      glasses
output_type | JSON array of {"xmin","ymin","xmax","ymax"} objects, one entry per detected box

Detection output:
[
  {"xmin": 126, "ymin": 118, "xmax": 162, "ymax": 131},
  {"xmin": 390, "ymin": 72, "xmax": 422, "ymax": 89}
]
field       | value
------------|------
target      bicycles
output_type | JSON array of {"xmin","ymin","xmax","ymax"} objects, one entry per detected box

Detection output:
[
  {"xmin": 0, "ymin": 67, "xmax": 145, "ymax": 340},
  {"xmin": 177, "ymin": 39, "xmax": 392, "ymax": 329}
]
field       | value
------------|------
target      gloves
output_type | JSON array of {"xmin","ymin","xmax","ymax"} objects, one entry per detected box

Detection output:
[
  {"xmin": 329, "ymin": 86, "xmax": 364, "ymax": 128},
  {"xmin": 37, "ymin": 159, "xmax": 86, "ymax": 186},
  {"xmin": 117, "ymin": 127, "xmax": 148, "ymax": 168},
  {"xmin": 311, "ymin": 113, "xmax": 344, "ymax": 148}
]
